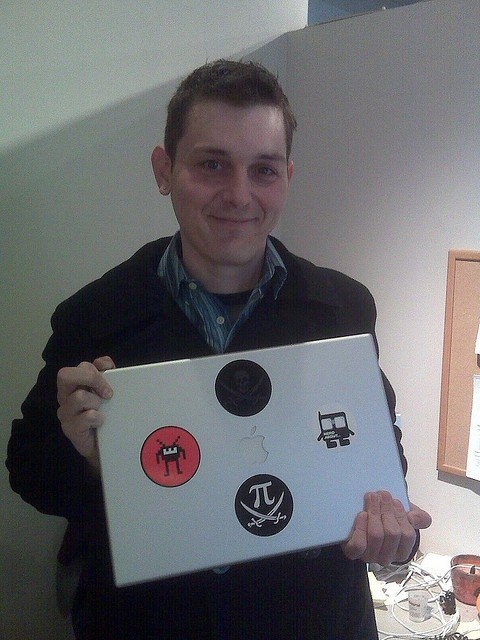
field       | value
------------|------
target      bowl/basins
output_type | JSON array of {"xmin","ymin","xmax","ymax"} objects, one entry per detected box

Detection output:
[{"xmin": 450, "ymin": 554, "xmax": 480, "ymax": 606}]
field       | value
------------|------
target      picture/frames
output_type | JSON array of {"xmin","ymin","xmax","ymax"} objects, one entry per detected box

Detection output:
[{"xmin": 436, "ymin": 250, "xmax": 480, "ymax": 480}]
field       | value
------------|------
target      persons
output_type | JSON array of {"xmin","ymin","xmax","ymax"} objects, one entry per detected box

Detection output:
[{"xmin": 6, "ymin": 57, "xmax": 432, "ymax": 639}]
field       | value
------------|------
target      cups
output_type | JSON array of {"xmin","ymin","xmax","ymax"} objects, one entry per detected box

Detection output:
[{"xmin": 408, "ymin": 588, "xmax": 429, "ymax": 622}]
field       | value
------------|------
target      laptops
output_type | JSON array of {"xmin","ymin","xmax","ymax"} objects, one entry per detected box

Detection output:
[{"xmin": 92, "ymin": 334, "xmax": 411, "ymax": 589}]
